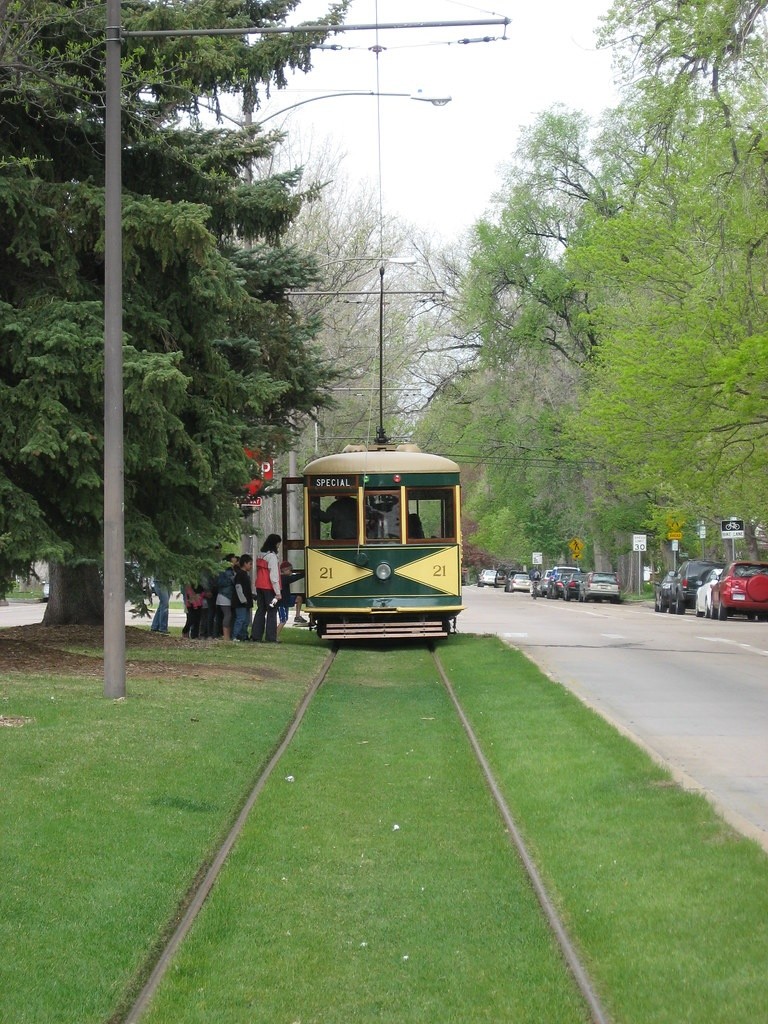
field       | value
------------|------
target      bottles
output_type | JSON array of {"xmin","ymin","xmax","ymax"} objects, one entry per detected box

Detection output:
[{"xmin": 269, "ymin": 596, "xmax": 278, "ymax": 608}]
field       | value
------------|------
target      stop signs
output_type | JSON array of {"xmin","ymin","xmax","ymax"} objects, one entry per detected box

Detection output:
[{"xmin": 233, "ymin": 444, "xmax": 274, "ymax": 496}]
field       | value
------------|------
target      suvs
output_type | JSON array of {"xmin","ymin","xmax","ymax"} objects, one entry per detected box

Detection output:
[
  {"xmin": 710, "ymin": 561, "xmax": 768, "ymax": 622},
  {"xmin": 496, "ymin": 569, "xmax": 528, "ymax": 592},
  {"xmin": 547, "ymin": 566, "xmax": 582, "ymax": 599},
  {"xmin": 668, "ymin": 558, "xmax": 727, "ymax": 615}
]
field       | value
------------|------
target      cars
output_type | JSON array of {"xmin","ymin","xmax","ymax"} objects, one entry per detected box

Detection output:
[
  {"xmin": 123, "ymin": 561, "xmax": 159, "ymax": 595},
  {"xmin": 41, "ymin": 577, "xmax": 50, "ymax": 602},
  {"xmin": 535, "ymin": 570, "xmax": 553, "ymax": 597},
  {"xmin": 508, "ymin": 574, "xmax": 533, "ymax": 593},
  {"xmin": 694, "ymin": 568, "xmax": 724, "ymax": 617},
  {"xmin": 578, "ymin": 571, "xmax": 621, "ymax": 604},
  {"xmin": 563, "ymin": 573, "xmax": 586, "ymax": 601},
  {"xmin": 654, "ymin": 569, "xmax": 679, "ymax": 613},
  {"xmin": 477, "ymin": 569, "xmax": 498, "ymax": 587}
]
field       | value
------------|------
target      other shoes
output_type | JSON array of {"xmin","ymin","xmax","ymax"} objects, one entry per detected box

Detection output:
[
  {"xmin": 158, "ymin": 630, "xmax": 170, "ymax": 636},
  {"xmin": 269, "ymin": 640, "xmax": 283, "ymax": 644},
  {"xmin": 151, "ymin": 629, "xmax": 159, "ymax": 633},
  {"xmin": 250, "ymin": 639, "xmax": 263, "ymax": 643}
]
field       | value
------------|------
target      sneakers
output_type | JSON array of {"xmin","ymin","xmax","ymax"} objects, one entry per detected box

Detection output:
[{"xmin": 294, "ymin": 616, "xmax": 307, "ymax": 622}]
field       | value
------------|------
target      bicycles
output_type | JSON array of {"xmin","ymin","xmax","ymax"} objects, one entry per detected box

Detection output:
[{"xmin": 531, "ymin": 581, "xmax": 537, "ymax": 598}]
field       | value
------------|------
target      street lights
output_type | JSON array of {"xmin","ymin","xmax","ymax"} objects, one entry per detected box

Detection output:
[
  {"xmin": 161, "ymin": 90, "xmax": 453, "ymax": 252},
  {"xmin": 240, "ymin": 254, "xmax": 418, "ymax": 596}
]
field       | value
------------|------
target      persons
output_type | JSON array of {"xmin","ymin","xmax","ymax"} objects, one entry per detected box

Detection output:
[
  {"xmin": 251, "ymin": 534, "xmax": 282, "ymax": 643},
  {"xmin": 529, "ymin": 566, "xmax": 541, "ymax": 596},
  {"xmin": 314, "ymin": 496, "xmax": 385, "ymax": 539},
  {"xmin": 277, "ymin": 561, "xmax": 307, "ymax": 637},
  {"xmin": 151, "ymin": 542, "xmax": 256, "ymax": 642}
]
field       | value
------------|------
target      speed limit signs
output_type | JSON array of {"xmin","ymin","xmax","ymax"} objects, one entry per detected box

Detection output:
[{"xmin": 632, "ymin": 533, "xmax": 647, "ymax": 552}]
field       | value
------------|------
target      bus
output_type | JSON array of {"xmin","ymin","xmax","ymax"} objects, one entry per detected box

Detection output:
[{"xmin": 303, "ymin": 436, "xmax": 468, "ymax": 639}]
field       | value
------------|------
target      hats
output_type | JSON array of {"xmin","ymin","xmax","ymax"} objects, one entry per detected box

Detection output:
[{"xmin": 280, "ymin": 561, "xmax": 292, "ymax": 571}]
